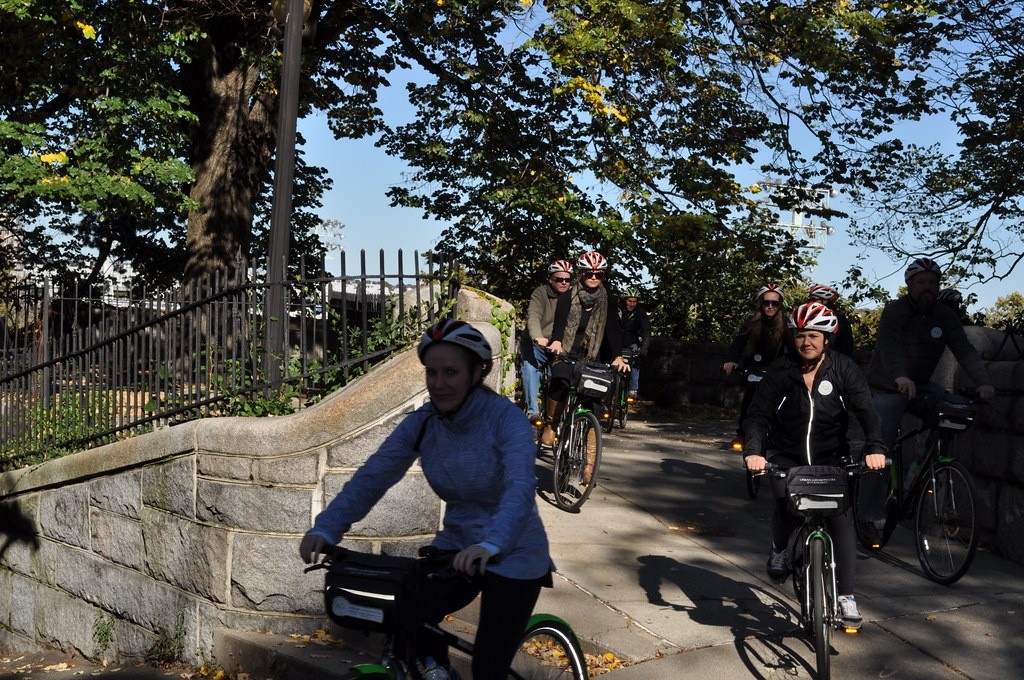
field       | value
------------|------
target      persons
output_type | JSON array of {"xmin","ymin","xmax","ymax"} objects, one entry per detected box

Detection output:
[
  {"xmin": 617, "ymin": 289, "xmax": 651, "ymax": 401},
  {"xmin": 518, "ymin": 252, "xmax": 631, "ymax": 487},
  {"xmin": 742, "ymin": 302, "xmax": 887, "ymax": 633},
  {"xmin": 808, "ymin": 284, "xmax": 855, "ymax": 356},
  {"xmin": 859, "ymin": 257, "xmax": 995, "ymax": 521},
  {"xmin": 938, "ymin": 290, "xmax": 976, "ymax": 326},
  {"xmin": 299, "ymin": 318, "xmax": 556, "ymax": 680},
  {"xmin": 722, "ymin": 284, "xmax": 797, "ymax": 442}
]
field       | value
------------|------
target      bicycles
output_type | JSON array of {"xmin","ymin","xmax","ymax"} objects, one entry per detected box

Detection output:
[
  {"xmin": 740, "ymin": 455, "xmax": 894, "ymax": 680},
  {"xmin": 719, "ymin": 363, "xmax": 768, "ymax": 499},
  {"xmin": 846, "ymin": 378, "xmax": 999, "ymax": 587},
  {"xmin": 303, "ymin": 544, "xmax": 589, "ymax": 680},
  {"xmin": 519, "ymin": 339, "xmax": 642, "ymax": 513}
]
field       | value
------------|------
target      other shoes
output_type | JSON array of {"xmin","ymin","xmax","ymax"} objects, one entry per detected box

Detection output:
[{"xmin": 629, "ymin": 390, "xmax": 641, "ymax": 401}]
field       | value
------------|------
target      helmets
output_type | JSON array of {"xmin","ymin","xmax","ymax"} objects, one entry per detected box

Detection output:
[
  {"xmin": 937, "ymin": 289, "xmax": 961, "ymax": 303},
  {"xmin": 548, "ymin": 260, "xmax": 574, "ymax": 275},
  {"xmin": 576, "ymin": 253, "xmax": 608, "ymax": 270},
  {"xmin": 906, "ymin": 258, "xmax": 942, "ymax": 283},
  {"xmin": 810, "ymin": 283, "xmax": 840, "ymax": 302},
  {"xmin": 620, "ymin": 287, "xmax": 641, "ymax": 298},
  {"xmin": 418, "ymin": 319, "xmax": 492, "ymax": 376},
  {"xmin": 787, "ymin": 302, "xmax": 838, "ymax": 334},
  {"xmin": 757, "ymin": 283, "xmax": 784, "ymax": 300}
]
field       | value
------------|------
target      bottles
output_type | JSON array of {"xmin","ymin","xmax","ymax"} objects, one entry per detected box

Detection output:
[
  {"xmin": 889, "ymin": 457, "xmax": 899, "ymax": 490},
  {"xmin": 422, "ymin": 656, "xmax": 450, "ymax": 680},
  {"xmin": 903, "ymin": 461, "xmax": 921, "ymax": 490}
]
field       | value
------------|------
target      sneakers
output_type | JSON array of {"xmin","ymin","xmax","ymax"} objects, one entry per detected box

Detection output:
[
  {"xmin": 858, "ymin": 521, "xmax": 882, "ymax": 549},
  {"xmin": 838, "ymin": 594, "xmax": 863, "ymax": 628},
  {"xmin": 767, "ymin": 539, "xmax": 789, "ymax": 584}
]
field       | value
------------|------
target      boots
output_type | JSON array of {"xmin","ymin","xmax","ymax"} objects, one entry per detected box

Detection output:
[
  {"xmin": 583, "ymin": 428, "xmax": 596, "ymax": 488},
  {"xmin": 541, "ymin": 395, "xmax": 563, "ymax": 448}
]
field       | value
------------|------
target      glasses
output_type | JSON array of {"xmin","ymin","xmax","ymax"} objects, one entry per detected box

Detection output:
[
  {"xmin": 760, "ymin": 300, "xmax": 780, "ymax": 306},
  {"xmin": 810, "ymin": 297, "xmax": 823, "ymax": 302},
  {"xmin": 550, "ymin": 276, "xmax": 571, "ymax": 283},
  {"xmin": 584, "ymin": 272, "xmax": 604, "ymax": 279}
]
